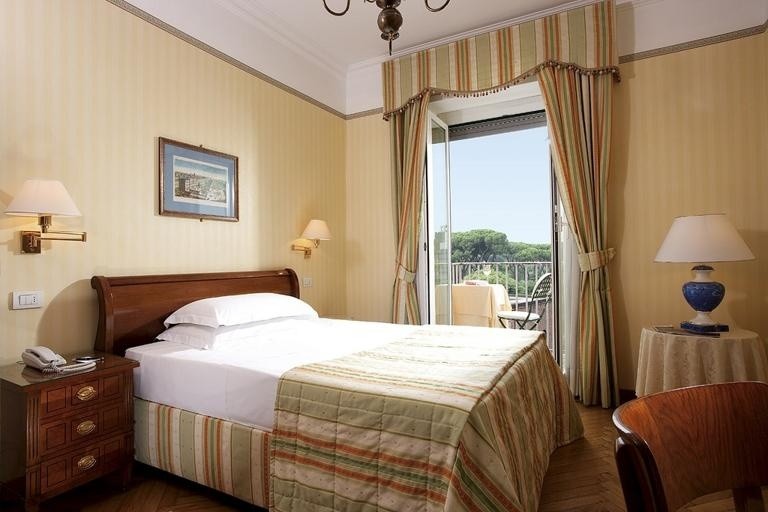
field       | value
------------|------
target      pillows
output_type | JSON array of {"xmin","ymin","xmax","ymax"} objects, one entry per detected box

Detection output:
[
  {"xmin": 155, "ymin": 316, "xmax": 322, "ymax": 351},
  {"xmin": 163, "ymin": 291, "xmax": 317, "ymax": 329}
]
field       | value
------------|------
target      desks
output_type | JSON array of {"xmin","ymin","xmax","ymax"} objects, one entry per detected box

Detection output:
[
  {"xmin": 634, "ymin": 323, "xmax": 767, "ymax": 400},
  {"xmin": 435, "ymin": 283, "xmax": 512, "ymax": 327}
]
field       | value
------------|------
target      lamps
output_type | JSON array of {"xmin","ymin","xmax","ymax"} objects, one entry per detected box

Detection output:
[
  {"xmin": 292, "ymin": 219, "xmax": 333, "ymax": 260},
  {"xmin": 652, "ymin": 214, "xmax": 757, "ymax": 333},
  {"xmin": 3, "ymin": 179, "xmax": 87, "ymax": 254}
]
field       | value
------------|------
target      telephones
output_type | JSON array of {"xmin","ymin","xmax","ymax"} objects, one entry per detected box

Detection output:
[{"xmin": 22, "ymin": 346, "xmax": 67, "ymax": 371}]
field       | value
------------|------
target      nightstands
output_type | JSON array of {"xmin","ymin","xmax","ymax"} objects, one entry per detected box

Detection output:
[{"xmin": 0, "ymin": 350, "xmax": 140, "ymax": 511}]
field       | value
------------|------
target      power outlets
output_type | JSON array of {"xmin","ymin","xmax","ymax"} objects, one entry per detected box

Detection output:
[
  {"xmin": 12, "ymin": 290, "xmax": 41, "ymax": 309},
  {"xmin": 303, "ymin": 277, "xmax": 313, "ymax": 288}
]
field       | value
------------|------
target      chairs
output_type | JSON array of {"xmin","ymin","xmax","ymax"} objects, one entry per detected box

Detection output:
[
  {"xmin": 497, "ymin": 273, "xmax": 552, "ymax": 330},
  {"xmin": 611, "ymin": 380, "xmax": 768, "ymax": 511}
]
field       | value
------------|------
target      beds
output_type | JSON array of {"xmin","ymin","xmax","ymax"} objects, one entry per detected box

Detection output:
[{"xmin": 90, "ymin": 267, "xmax": 584, "ymax": 512}]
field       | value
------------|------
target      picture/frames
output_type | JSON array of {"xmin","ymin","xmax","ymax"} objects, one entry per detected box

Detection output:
[{"xmin": 158, "ymin": 136, "xmax": 240, "ymax": 223}]
